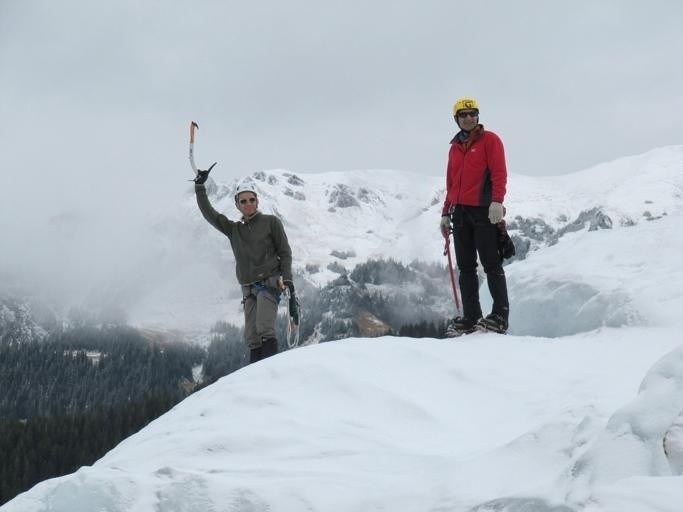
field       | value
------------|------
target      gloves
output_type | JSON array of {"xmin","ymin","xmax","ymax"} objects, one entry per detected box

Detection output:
[
  {"xmin": 283, "ymin": 281, "xmax": 295, "ymax": 293},
  {"xmin": 194, "ymin": 169, "xmax": 208, "ymax": 184},
  {"xmin": 488, "ymin": 202, "xmax": 504, "ymax": 224},
  {"xmin": 440, "ymin": 215, "xmax": 451, "ymax": 236}
]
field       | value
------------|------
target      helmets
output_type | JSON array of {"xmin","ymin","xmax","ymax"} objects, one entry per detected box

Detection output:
[
  {"xmin": 452, "ymin": 97, "xmax": 479, "ymax": 116},
  {"xmin": 235, "ymin": 183, "xmax": 258, "ymax": 199}
]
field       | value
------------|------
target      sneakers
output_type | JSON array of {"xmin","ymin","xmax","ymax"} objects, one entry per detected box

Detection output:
[
  {"xmin": 447, "ymin": 316, "xmax": 482, "ymax": 332},
  {"xmin": 478, "ymin": 313, "xmax": 508, "ymax": 333}
]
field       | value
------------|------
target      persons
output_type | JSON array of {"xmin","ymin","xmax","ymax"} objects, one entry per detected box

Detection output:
[
  {"xmin": 440, "ymin": 99, "xmax": 510, "ymax": 335},
  {"xmin": 194, "ymin": 169, "xmax": 292, "ymax": 363}
]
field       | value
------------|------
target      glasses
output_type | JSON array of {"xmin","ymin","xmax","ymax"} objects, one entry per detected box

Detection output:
[
  {"xmin": 240, "ymin": 198, "xmax": 255, "ymax": 204},
  {"xmin": 458, "ymin": 111, "xmax": 477, "ymax": 117}
]
